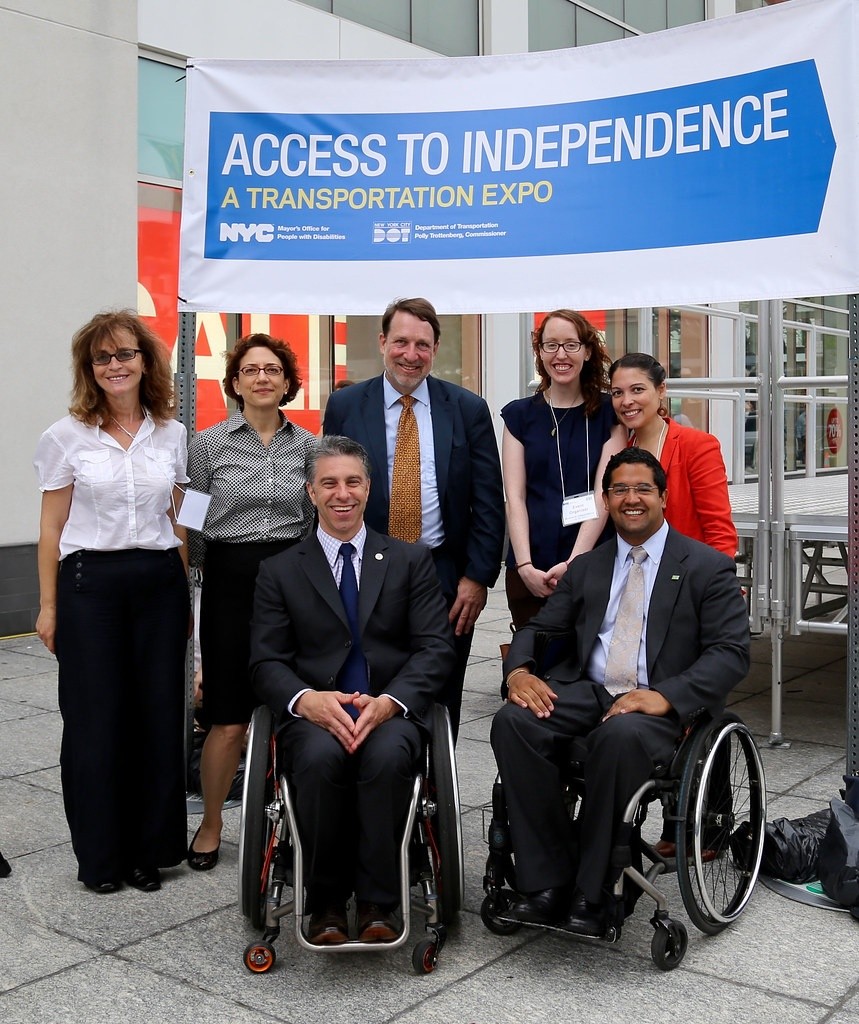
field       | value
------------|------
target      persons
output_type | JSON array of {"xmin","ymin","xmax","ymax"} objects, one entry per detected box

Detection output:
[
  {"xmin": 246, "ymin": 429, "xmax": 460, "ymax": 946},
  {"xmin": 35, "ymin": 309, "xmax": 192, "ymax": 896},
  {"xmin": 321, "ymin": 297, "xmax": 507, "ymax": 753},
  {"xmin": 607, "ymin": 352, "xmax": 744, "ymax": 865},
  {"xmin": 488, "ymin": 447, "xmax": 753, "ymax": 940},
  {"xmin": 180, "ymin": 332, "xmax": 319, "ymax": 872},
  {"xmin": 500, "ymin": 309, "xmax": 630, "ymax": 631}
]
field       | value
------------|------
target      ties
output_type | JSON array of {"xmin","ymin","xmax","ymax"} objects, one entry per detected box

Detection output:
[
  {"xmin": 602, "ymin": 545, "xmax": 648, "ymax": 697},
  {"xmin": 387, "ymin": 395, "xmax": 422, "ymax": 544},
  {"xmin": 337, "ymin": 543, "xmax": 369, "ymax": 723}
]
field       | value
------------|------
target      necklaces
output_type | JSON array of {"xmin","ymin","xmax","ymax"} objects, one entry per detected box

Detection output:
[
  {"xmin": 109, "ymin": 418, "xmax": 136, "ymax": 437},
  {"xmin": 631, "ymin": 420, "xmax": 666, "ymax": 459},
  {"xmin": 547, "ymin": 391, "xmax": 579, "ymax": 437}
]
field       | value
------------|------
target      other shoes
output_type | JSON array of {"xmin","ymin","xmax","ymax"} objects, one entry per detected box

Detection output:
[
  {"xmin": 354, "ymin": 899, "xmax": 400, "ymax": 942},
  {"xmin": 307, "ymin": 900, "xmax": 350, "ymax": 941}
]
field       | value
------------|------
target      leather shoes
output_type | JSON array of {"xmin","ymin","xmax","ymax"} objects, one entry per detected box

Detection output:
[
  {"xmin": 691, "ymin": 850, "xmax": 722, "ymax": 866},
  {"xmin": 82, "ymin": 873, "xmax": 116, "ymax": 894},
  {"xmin": 653, "ymin": 839, "xmax": 685, "ymax": 858},
  {"xmin": 513, "ymin": 888, "xmax": 562, "ymax": 924},
  {"xmin": 122, "ymin": 865, "xmax": 161, "ymax": 892},
  {"xmin": 187, "ymin": 824, "xmax": 221, "ymax": 871},
  {"xmin": 565, "ymin": 890, "xmax": 604, "ymax": 936}
]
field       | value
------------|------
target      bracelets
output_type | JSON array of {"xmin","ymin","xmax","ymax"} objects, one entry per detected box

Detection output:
[
  {"xmin": 506, "ymin": 670, "xmax": 528, "ymax": 687},
  {"xmin": 517, "ymin": 562, "xmax": 532, "ymax": 569},
  {"xmin": 566, "ymin": 561, "xmax": 569, "ymax": 566}
]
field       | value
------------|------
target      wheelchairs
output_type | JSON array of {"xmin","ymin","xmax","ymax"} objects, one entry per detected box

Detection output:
[
  {"xmin": 477, "ymin": 620, "xmax": 773, "ymax": 973},
  {"xmin": 236, "ymin": 680, "xmax": 468, "ymax": 976}
]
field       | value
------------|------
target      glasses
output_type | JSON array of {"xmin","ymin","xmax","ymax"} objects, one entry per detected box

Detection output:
[
  {"xmin": 89, "ymin": 348, "xmax": 145, "ymax": 365},
  {"xmin": 607, "ymin": 484, "xmax": 662, "ymax": 496},
  {"xmin": 236, "ymin": 364, "xmax": 286, "ymax": 376},
  {"xmin": 538, "ymin": 341, "xmax": 586, "ymax": 353}
]
